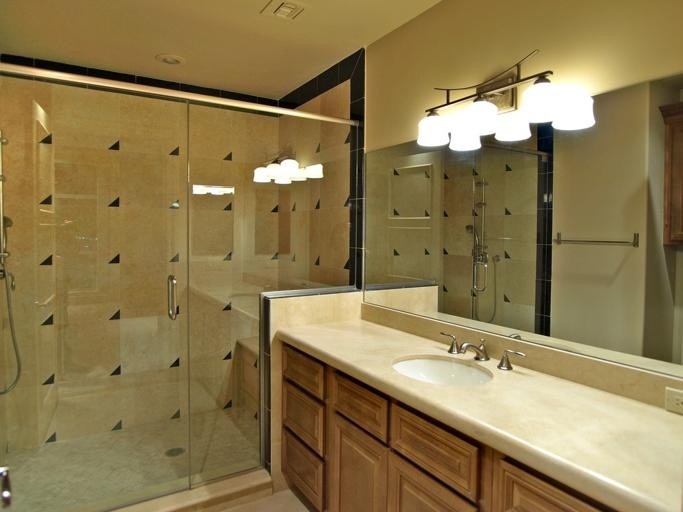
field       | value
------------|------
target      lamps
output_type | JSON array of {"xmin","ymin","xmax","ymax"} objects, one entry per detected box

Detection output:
[
  {"xmin": 193, "ymin": 185, "xmax": 235, "ymax": 196},
  {"xmin": 253, "ymin": 153, "xmax": 325, "ymax": 185},
  {"xmin": 417, "ymin": 50, "xmax": 596, "ymax": 152}
]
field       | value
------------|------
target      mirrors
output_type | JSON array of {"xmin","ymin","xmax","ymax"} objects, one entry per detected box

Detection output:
[{"xmin": 364, "ymin": 73, "xmax": 683, "ymax": 365}]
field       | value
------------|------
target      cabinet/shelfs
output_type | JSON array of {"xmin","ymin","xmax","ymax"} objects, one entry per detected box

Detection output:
[
  {"xmin": 281, "ymin": 343, "xmax": 325, "ymax": 512},
  {"xmin": 332, "ymin": 373, "xmax": 478, "ymax": 512},
  {"xmin": 498, "ymin": 459, "xmax": 606, "ymax": 512},
  {"xmin": 232, "ymin": 335, "xmax": 259, "ymax": 449}
]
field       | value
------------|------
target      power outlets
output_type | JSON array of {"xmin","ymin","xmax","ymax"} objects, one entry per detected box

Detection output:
[{"xmin": 664, "ymin": 387, "xmax": 683, "ymax": 415}]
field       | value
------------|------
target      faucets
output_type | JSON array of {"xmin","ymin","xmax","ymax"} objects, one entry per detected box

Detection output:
[
  {"xmin": 459, "ymin": 337, "xmax": 488, "ymax": 361},
  {"xmin": 508, "ymin": 334, "xmax": 521, "ymax": 340}
]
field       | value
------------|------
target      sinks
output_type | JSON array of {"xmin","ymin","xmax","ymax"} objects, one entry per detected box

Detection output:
[{"xmin": 392, "ymin": 355, "xmax": 493, "ymax": 387}]
